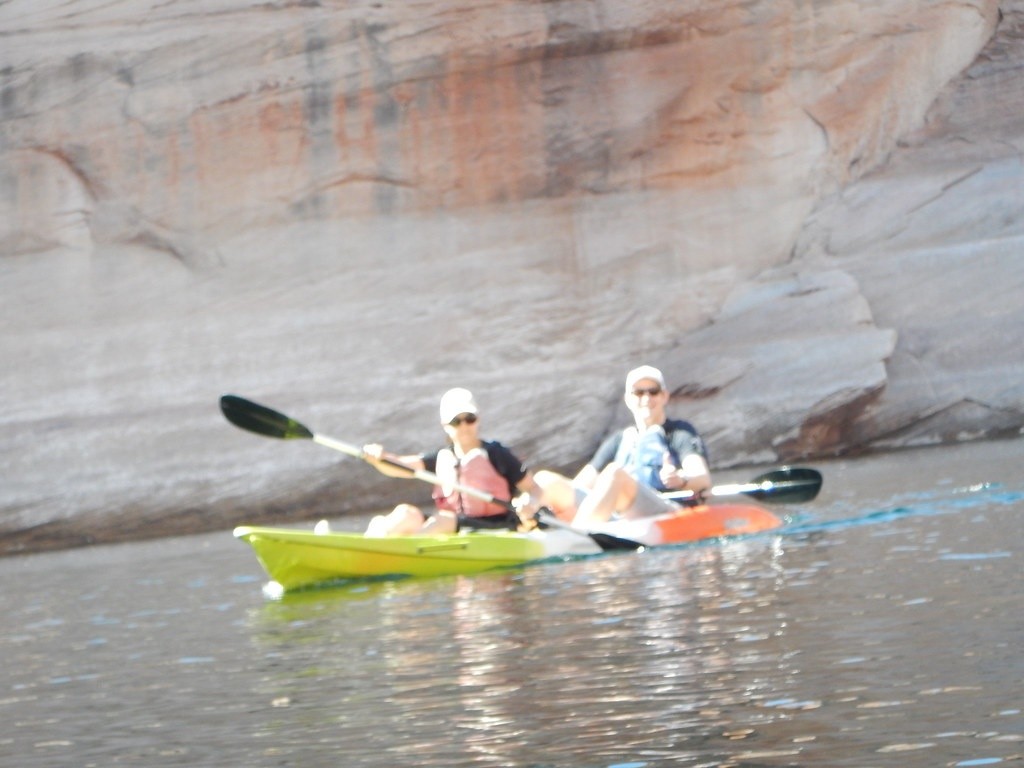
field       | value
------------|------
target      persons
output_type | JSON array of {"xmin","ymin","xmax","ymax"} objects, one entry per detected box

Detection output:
[
  {"xmin": 316, "ymin": 387, "xmax": 547, "ymax": 537},
  {"xmin": 515, "ymin": 366, "xmax": 714, "ymax": 534}
]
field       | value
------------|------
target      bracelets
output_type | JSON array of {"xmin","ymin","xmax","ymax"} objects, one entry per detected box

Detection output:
[{"xmin": 681, "ymin": 474, "xmax": 687, "ymax": 490}]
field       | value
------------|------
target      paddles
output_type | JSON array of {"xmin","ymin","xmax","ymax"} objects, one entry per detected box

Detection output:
[
  {"xmin": 216, "ymin": 391, "xmax": 647, "ymax": 550},
  {"xmin": 661, "ymin": 468, "xmax": 824, "ymax": 503}
]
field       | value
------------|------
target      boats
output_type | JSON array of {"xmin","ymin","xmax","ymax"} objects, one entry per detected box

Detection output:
[{"xmin": 233, "ymin": 504, "xmax": 783, "ymax": 592}]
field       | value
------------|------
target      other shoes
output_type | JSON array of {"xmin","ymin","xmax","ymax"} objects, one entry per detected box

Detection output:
[
  {"xmin": 365, "ymin": 518, "xmax": 387, "ymax": 536},
  {"xmin": 314, "ymin": 520, "xmax": 330, "ymax": 533}
]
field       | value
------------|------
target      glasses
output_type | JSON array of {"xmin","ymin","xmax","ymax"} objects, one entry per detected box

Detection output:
[
  {"xmin": 631, "ymin": 388, "xmax": 660, "ymax": 398},
  {"xmin": 449, "ymin": 414, "xmax": 476, "ymax": 426}
]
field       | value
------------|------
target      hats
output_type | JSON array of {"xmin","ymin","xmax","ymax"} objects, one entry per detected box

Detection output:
[
  {"xmin": 440, "ymin": 388, "xmax": 477, "ymax": 424},
  {"xmin": 625, "ymin": 366, "xmax": 665, "ymax": 394}
]
